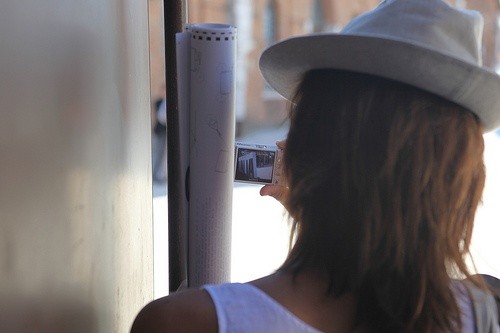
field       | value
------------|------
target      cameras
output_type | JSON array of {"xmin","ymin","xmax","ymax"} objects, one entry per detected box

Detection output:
[{"xmin": 233, "ymin": 143, "xmax": 288, "ymax": 187}]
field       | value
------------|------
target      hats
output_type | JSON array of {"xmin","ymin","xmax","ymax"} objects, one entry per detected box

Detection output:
[{"xmin": 259, "ymin": 0, "xmax": 500, "ymax": 135}]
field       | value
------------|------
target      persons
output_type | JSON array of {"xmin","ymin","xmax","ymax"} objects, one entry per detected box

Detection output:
[{"xmin": 130, "ymin": 1, "xmax": 500, "ymax": 333}]
